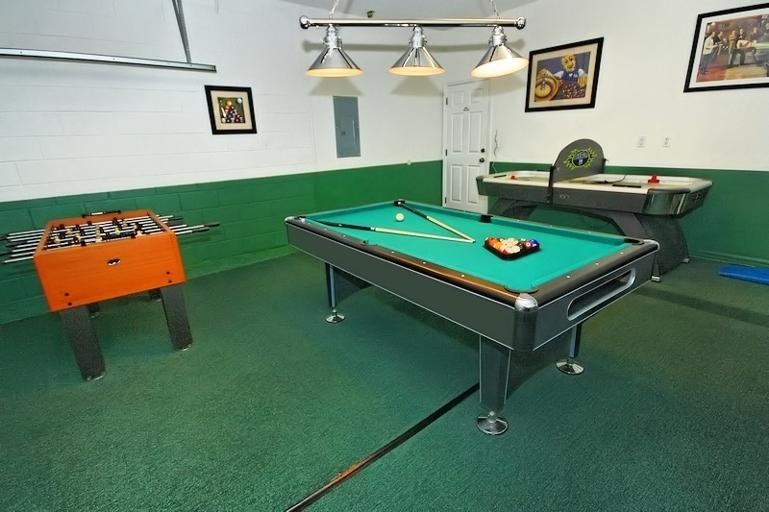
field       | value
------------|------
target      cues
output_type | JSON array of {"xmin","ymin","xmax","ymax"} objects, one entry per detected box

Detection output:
[
  {"xmin": 394, "ymin": 199, "xmax": 475, "ymax": 242},
  {"xmin": 295, "ymin": 216, "xmax": 474, "ymax": 244}
]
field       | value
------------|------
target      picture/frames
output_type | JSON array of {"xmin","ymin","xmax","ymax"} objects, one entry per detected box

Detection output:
[
  {"xmin": 682, "ymin": 2, "xmax": 768, "ymax": 93},
  {"xmin": 524, "ymin": 33, "xmax": 605, "ymax": 112},
  {"xmin": 204, "ymin": 82, "xmax": 258, "ymax": 135}
]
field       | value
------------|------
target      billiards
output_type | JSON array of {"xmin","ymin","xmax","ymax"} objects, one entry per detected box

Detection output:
[
  {"xmin": 485, "ymin": 237, "xmax": 539, "ymax": 255},
  {"xmin": 395, "ymin": 213, "xmax": 405, "ymax": 222},
  {"xmin": 221, "ymin": 101, "xmax": 244, "ymax": 123},
  {"xmin": 237, "ymin": 99, "xmax": 243, "ymax": 103}
]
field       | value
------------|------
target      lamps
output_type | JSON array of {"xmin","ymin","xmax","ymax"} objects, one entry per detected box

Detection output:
[
  {"xmin": 388, "ymin": 25, "xmax": 446, "ymax": 78},
  {"xmin": 471, "ymin": 25, "xmax": 528, "ymax": 80},
  {"xmin": 305, "ymin": 27, "xmax": 364, "ymax": 81}
]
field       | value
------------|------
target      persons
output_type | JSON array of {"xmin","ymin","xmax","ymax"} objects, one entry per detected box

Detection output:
[
  {"xmin": 764, "ymin": 22, "xmax": 769, "ymax": 44},
  {"xmin": 712, "ymin": 31, "xmax": 726, "ymax": 64},
  {"xmin": 749, "ymin": 27, "xmax": 762, "ymax": 44},
  {"xmin": 728, "ymin": 30, "xmax": 737, "ymax": 65},
  {"xmin": 736, "ymin": 28, "xmax": 747, "ymax": 64},
  {"xmin": 699, "ymin": 31, "xmax": 717, "ymax": 76}
]
